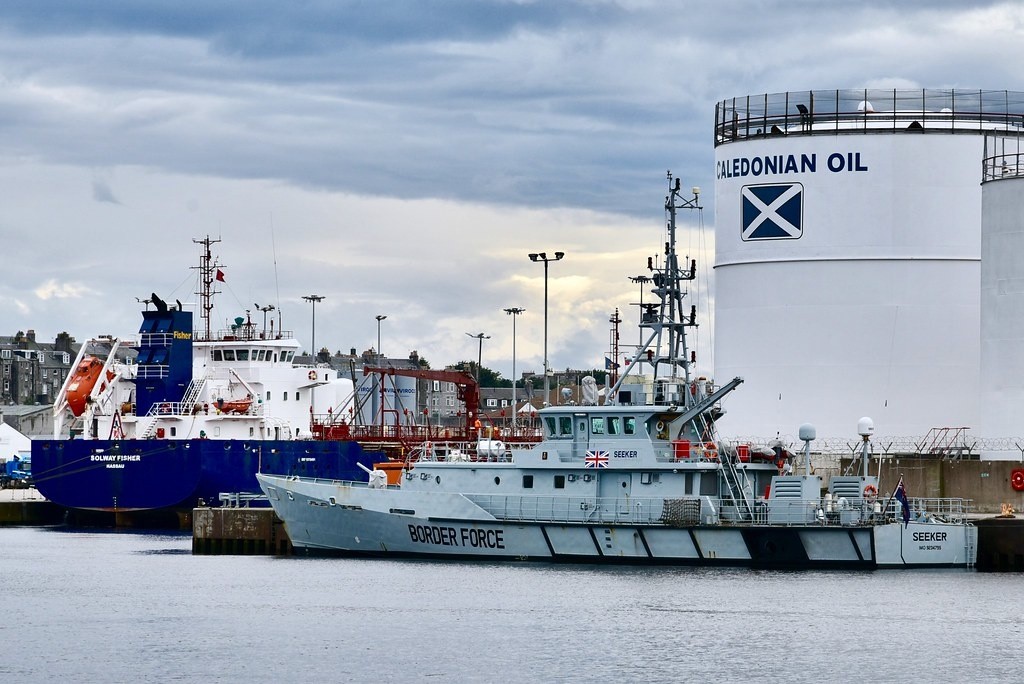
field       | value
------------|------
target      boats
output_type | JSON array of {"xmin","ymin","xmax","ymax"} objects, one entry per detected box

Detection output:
[
  {"xmin": 26, "ymin": 233, "xmax": 392, "ymax": 509},
  {"xmin": 253, "ymin": 167, "xmax": 979, "ymax": 571}
]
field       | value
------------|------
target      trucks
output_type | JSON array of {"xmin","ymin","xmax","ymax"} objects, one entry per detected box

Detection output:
[{"xmin": 0, "ymin": 458, "xmax": 34, "ymax": 489}]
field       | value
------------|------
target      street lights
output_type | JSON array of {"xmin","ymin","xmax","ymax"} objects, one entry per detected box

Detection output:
[
  {"xmin": 526, "ymin": 251, "xmax": 566, "ymax": 407},
  {"xmin": 503, "ymin": 305, "xmax": 526, "ymax": 432},
  {"xmin": 465, "ymin": 329, "xmax": 492, "ymax": 386},
  {"xmin": 300, "ymin": 293, "xmax": 329, "ymax": 368},
  {"xmin": 373, "ymin": 313, "xmax": 387, "ymax": 369}
]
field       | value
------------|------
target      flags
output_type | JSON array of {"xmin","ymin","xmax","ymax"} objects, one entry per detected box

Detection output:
[{"xmin": 892, "ymin": 477, "xmax": 911, "ymax": 529}]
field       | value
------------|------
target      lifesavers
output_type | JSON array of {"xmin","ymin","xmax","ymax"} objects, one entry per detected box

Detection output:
[
  {"xmin": 704, "ymin": 442, "xmax": 717, "ymax": 457},
  {"xmin": 1012, "ymin": 472, "xmax": 1024, "ymax": 491},
  {"xmin": 162, "ymin": 404, "xmax": 171, "ymax": 413},
  {"xmin": 864, "ymin": 485, "xmax": 877, "ymax": 504},
  {"xmin": 308, "ymin": 370, "xmax": 316, "ymax": 380}
]
